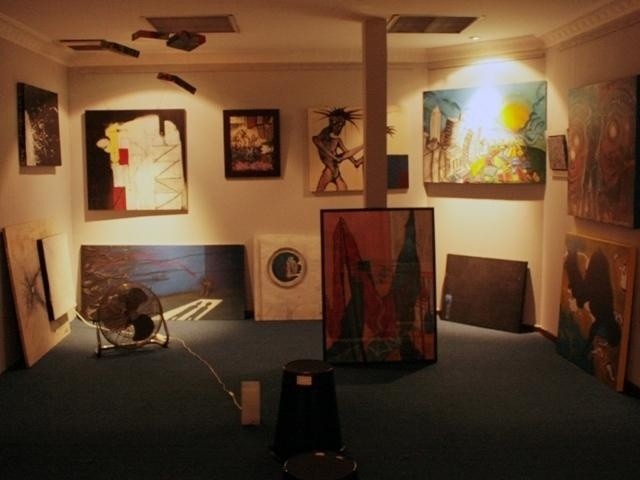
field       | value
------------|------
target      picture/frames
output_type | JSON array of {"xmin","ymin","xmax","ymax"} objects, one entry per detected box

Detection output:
[
  {"xmin": 319, "ymin": 208, "xmax": 437, "ymax": 366},
  {"xmin": 223, "ymin": 108, "xmax": 280, "ymax": 177}
]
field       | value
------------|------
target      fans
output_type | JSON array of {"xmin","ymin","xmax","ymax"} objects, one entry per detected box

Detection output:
[{"xmin": 94, "ymin": 282, "xmax": 171, "ymax": 355}]
